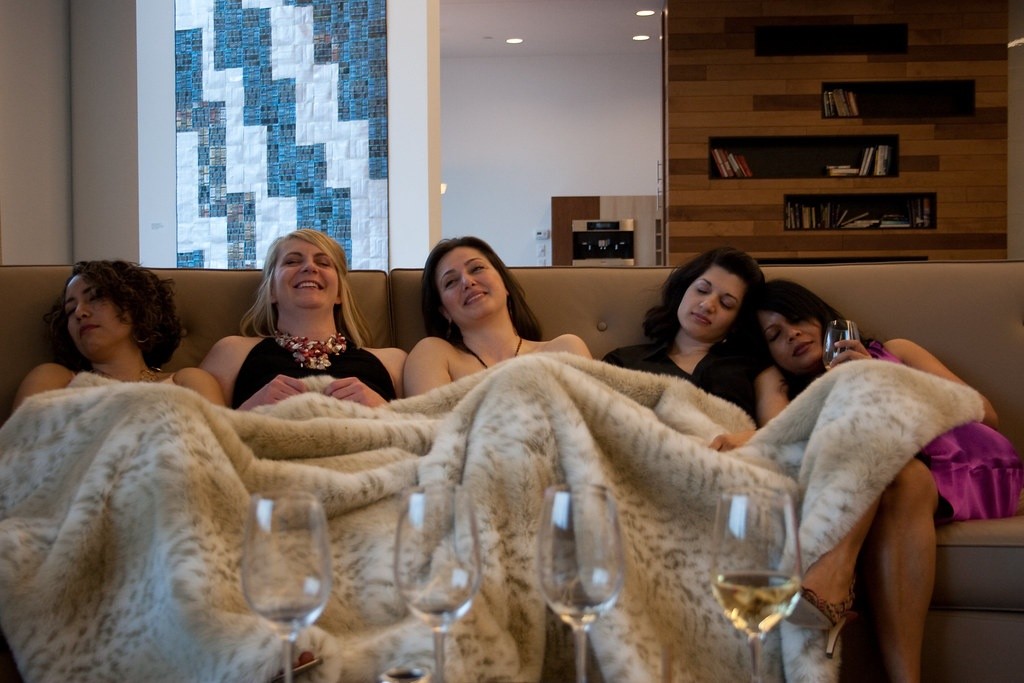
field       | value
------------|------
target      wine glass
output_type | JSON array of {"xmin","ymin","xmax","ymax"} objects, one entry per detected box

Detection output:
[
  {"xmin": 240, "ymin": 490, "xmax": 333, "ymax": 683},
  {"xmin": 708, "ymin": 487, "xmax": 805, "ymax": 683},
  {"xmin": 536, "ymin": 485, "xmax": 629, "ymax": 683},
  {"xmin": 391, "ymin": 489, "xmax": 490, "ymax": 683}
]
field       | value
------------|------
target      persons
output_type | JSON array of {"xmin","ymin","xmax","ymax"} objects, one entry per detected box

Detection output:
[
  {"xmin": 11, "ymin": 261, "xmax": 322, "ymax": 678},
  {"xmin": 601, "ymin": 248, "xmax": 789, "ymax": 452},
  {"xmin": 198, "ymin": 230, "xmax": 407, "ymax": 412},
  {"xmin": 756, "ymin": 279, "xmax": 1024, "ymax": 683},
  {"xmin": 403, "ymin": 237, "xmax": 593, "ymax": 397}
]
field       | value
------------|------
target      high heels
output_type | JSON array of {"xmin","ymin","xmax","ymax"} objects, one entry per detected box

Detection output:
[{"xmin": 769, "ymin": 576, "xmax": 856, "ymax": 659}]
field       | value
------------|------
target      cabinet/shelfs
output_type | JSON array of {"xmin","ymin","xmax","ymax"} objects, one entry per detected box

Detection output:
[{"xmin": 660, "ymin": 0, "xmax": 1009, "ymax": 261}]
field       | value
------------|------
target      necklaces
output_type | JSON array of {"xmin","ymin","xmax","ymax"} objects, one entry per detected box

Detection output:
[
  {"xmin": 272, "ymin": 329, "xmax": 347, "ymax": 370},
  {"xmin": 94, "ymin": 366, "xmax": 159, "ymax": 383},
  {"xmin": 471, "ymin": 337, "xmax": 523, "ymax": 368}
]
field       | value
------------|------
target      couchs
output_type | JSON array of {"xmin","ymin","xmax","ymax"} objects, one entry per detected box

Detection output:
[{"xmin": 389, "ymin": 260, "xmax": 1024, "ymax": 682}]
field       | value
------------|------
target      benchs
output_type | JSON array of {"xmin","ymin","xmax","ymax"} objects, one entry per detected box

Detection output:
[{"xmin": 0, "ymin": 264, "xmax": 396, "ymax": 429}]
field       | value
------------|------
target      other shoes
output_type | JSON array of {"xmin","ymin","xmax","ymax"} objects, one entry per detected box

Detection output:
[
  {"xmin": 379, "ymin": 665, "xmax": 431, "ymax": 683},
  {"xmin": 271, "ymin": 646, "xmax": 323, "ymax": 682}
]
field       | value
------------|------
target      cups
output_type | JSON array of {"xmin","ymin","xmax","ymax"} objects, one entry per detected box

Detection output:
[{"xmin": 821, "ymin": 320, "xmax": 860, "ymax": 371}]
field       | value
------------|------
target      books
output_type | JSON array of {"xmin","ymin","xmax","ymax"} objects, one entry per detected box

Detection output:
[
  {"xmin": 711, "ymin": 145, "xmax": 753, "ymax": 177},
  {"xmin": 785, "ymin": 199, "xmax": 931, "ymax": 231},
  {"xmin": 827, "ymin": 146, "xmax": 891, "ymax": 176},
  {"xmin": 824, "ymin": 88, "xmax": 859, "ymax": 116}
]
